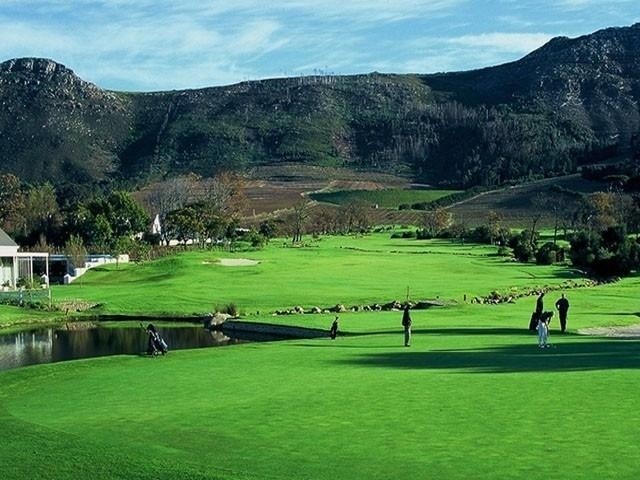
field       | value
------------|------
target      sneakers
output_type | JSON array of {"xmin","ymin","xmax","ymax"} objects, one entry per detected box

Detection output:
[{"xmin": 538, "ymin": 345, "xmax": 551, "ymax": 349}]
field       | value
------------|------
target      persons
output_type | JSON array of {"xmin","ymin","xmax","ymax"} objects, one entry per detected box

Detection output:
[
  {"xmin": 555, "ymin": 294, "xmax": 570, "ymax": 334},
  {"xmin": 145, "ymin": 324, "xmax": 160, "ymax": 357},
  {"xmin": 331, "ymin": 316, "xmax": 338, "ymax": 340},
  {"xmin": 401, "ymin": 302, "xmax": 414, "ymax": 348},
  {"xmin": 535, "ymin": 293, "xmax": 546, "ymax": 322},
  {"xmin": 538, "ymin": 310, "xmax": 553, "ymax": 349}
]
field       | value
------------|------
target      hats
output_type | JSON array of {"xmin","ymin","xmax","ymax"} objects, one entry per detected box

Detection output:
[
  {"xmin": 551, "ymin": 311, "xmax": 555, "ymax": 317},
  {"xmin": 407, "ymin": 302, "xmax": 413, "ymax": 307}
]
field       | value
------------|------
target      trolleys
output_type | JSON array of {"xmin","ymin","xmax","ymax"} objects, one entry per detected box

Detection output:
[{"xmin": 139, "ymin": 323, "xmax": 168, "ymax": 356}]
